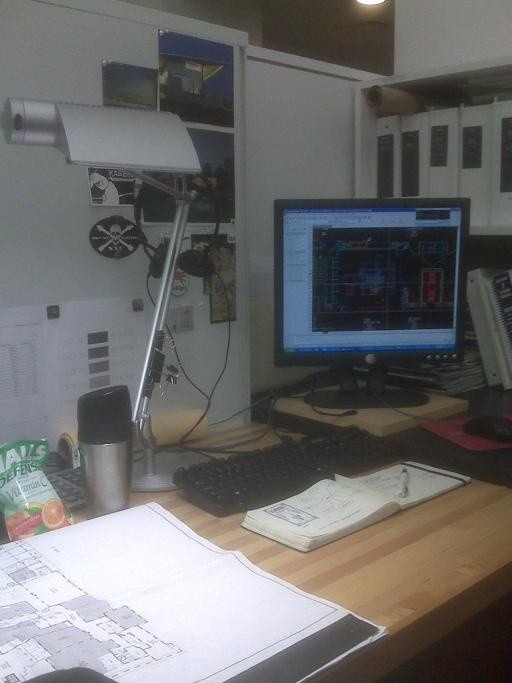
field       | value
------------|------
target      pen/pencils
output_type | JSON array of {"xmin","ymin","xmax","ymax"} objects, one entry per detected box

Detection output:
[{"xmin": 398, "ymin": 468, "xmax": 408, "ymax": 498}]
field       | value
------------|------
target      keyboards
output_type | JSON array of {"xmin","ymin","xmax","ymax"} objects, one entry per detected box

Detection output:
[{"xmin": 172, "ymin": 423, "xmax": 414, "ymax": 517}]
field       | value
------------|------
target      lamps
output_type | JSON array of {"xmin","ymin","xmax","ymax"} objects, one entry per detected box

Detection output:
[
  {"xmin": 6, "ymin": 100, "xmax": 210, "ymax": 493},
  {"xmin": 354, "ymin": 0, "xmax": 385, "ymax": 7}
]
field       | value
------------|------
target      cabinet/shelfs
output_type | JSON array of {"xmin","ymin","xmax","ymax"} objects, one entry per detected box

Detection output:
[{"xmin": 352, "ymin": 55, "xmax": 512, "ymax": 238}]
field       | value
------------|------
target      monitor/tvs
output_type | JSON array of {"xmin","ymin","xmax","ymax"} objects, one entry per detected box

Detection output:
[{"xmin": 272, "ymin": 196, "xmax": 469, "ymax": 410}]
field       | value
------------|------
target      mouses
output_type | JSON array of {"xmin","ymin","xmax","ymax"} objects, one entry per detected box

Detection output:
[{"xmin": 463, "ymin": 417, "xmax": 512, "ymax": 443}]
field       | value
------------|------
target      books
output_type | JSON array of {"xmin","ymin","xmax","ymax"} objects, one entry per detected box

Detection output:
[
  {"xmin": 240, "ymin": 459, "xmax": 472, "ymax": 553},
  {"xmin": 388, "ymin": 268, "xmax": 511, "ymax": 394}
]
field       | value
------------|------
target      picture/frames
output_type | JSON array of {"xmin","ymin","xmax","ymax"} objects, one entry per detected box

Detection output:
[
  {"xmin": 90, "ymin": 216, "xmax": 138, "ymax": 258},
  {"xmin": 157, "ymin": 29, "xmax": 234, "ymax": 128},
  {"xmin": 143, "ymin": 127, "xmax": 236, "ymax": 226},
  {"xmin": 101, "ymin": 59, "xmax": 160, "ymax": 109},
  {"xmin": 86, "ymin": 165, "xmax": 140, "ymax": 208}
]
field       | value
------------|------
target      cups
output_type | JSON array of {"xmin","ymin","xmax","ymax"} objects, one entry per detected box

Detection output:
[{"xmin": 79, "ymin": 385, "xmax": 134, "ymax": 520}]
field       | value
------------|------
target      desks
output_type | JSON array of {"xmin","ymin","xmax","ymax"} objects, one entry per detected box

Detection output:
[{"xmin": 33, "ymin": 379, "xmax": 509, "ymax": 681}]
